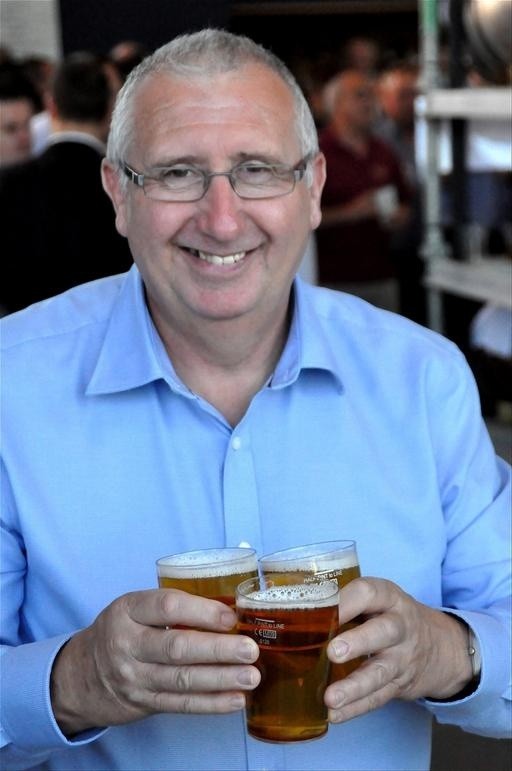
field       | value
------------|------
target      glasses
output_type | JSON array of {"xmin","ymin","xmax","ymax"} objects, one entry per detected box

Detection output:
[{"xmin": 117, "ymin": 152, "xmax": 312, "ymax": 202}]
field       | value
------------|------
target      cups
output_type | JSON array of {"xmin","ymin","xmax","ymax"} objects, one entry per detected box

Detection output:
[
  {"xmin": 234, "ymin": 573, "xmax": 341, "ymax": 744},
  {"xmin": 258, "ymin": 540, "xmax": 369, "ymax": 685},
  {"xmin": 156, "ymin": 548, "xmax": 258, "ymax": 696}
]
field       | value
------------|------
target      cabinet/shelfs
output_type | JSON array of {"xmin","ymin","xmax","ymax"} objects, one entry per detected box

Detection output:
[{"xmin": 411, "ymin": 1, "xmax": 512, "ymax": 335}]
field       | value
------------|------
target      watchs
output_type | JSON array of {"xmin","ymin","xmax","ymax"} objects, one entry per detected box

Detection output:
[{"xmin": 468, "ymin": 628, "xmax": 483, "ymax": 677}]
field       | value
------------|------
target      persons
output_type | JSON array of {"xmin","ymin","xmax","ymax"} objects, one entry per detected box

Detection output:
[
  {"xmin": 292, "ymin": 36, "xmax": 512, "ymax": 422},
  {"xmin": 0, "ymin": 29, "xmax": 512, "ymax": 771},
  {"xmin": 1, "ymin": 39, "xmax": 150, "ymax": 318}
]
